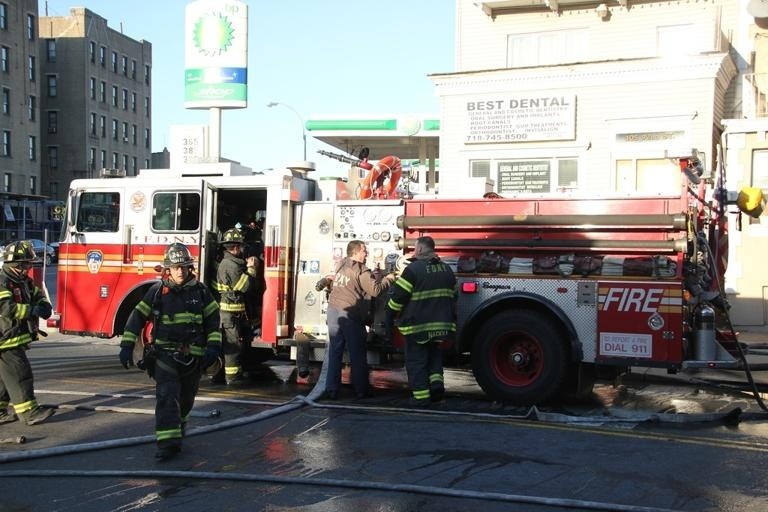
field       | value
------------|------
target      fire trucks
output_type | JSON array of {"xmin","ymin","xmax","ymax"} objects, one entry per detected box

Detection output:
[{"xmin": 47, "ymin": 153, "xmax": 736, "ymax": 403}]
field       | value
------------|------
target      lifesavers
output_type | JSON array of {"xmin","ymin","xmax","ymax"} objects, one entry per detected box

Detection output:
[{"xmin": 360, "ymin": 156, "xmax": 402, "ymax": 199}]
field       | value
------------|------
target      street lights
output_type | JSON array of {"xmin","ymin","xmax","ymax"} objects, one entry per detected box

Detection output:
[{"xmin": 263, "ymin": 100, "xmax": 310, "ymax": 164}]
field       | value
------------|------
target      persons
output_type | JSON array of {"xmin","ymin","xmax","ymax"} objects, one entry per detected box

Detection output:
[
  {"xmin": 208, "ymin": 227, "xmax": 260, "ymax": 388},
  {"xmin": 383, "ymin": 235, "xmax": 461, "ymax": 404},
  {"xmin": 324, "ymin": 238, "xmax": 399, "ymax": 402},
  {"xmin": 0, "ymin": 240, "xmax": 57, "ymax": 428},
  {"xmin": 116, "ymin": 242, "xmax": 220, "ymax": 462}
]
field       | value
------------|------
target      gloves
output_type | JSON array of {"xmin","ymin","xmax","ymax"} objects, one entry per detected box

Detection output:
[
  {"xmin": 40, "ymin": 301, "xmax": 53, "ymax": 319},
  {"xmin": 31, "ymin": 305, "xmax": 50, "ymax": 321},
  {"xmin": 117, "ymin": 348, "xmax": 138, "ymax": 371},
  {"xmin": 202, "ymin": 345, "xmax": 222, "ymax": 366}
]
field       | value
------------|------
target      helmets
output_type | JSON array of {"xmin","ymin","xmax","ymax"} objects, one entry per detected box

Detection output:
[
  {"xmin": 216, "ymin": 228, "xmax": 244, "ymax": 246},
  {"xmin": 161, "ymin": 241, "xmax": 197, "ymax": 269},
  {"xmin": 3, "ymin": 240, "xmax": 43, "ymax": 264}
]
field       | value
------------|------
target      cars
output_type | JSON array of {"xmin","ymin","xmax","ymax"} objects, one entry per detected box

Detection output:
[
  {"xmin": 46, "ymin": 242, "xmax": 58, "ymax": 258},
  {"xmin": 5, "ymin": 237, "xmax": 58, "ymax": 267}
]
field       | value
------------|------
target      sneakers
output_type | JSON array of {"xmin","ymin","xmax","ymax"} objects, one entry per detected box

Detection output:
[
  {"xmin": 22, "ymin": 404, "xmax": 54, "ymax": 427},
  {"xmin": 160, "ymin": 440, "xmax": 182, "ymax": 461},
  {"xmin": 0, "ymin": 409, "xmax": 17, "ymax": 426},
  {"xmin": 224, "ymin": 376, "xmax": 251, "ymax": 387}
]
